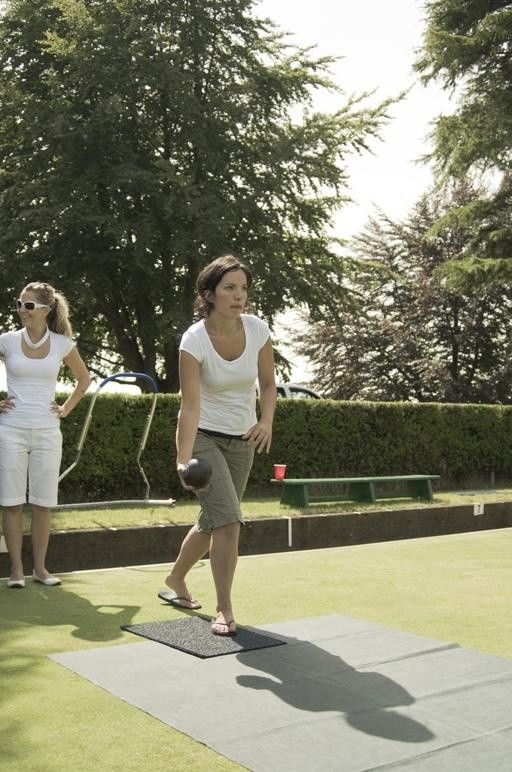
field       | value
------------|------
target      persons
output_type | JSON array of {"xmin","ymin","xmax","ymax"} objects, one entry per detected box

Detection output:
[
  {"xmin": 0, "ymin": 282, "xmax": 92, "ymax": 588},
  {"xmin": 158, "ymin": 255, "xmax": 277, "ymax": 636}
]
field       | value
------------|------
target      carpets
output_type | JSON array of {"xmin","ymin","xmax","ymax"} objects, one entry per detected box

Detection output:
[{"xmin": 121, "ymin": 616, "xmax": 287, "ymax": 659}]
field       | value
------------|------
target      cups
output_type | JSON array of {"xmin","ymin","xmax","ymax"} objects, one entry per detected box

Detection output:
[{"xmin": 273, "ymin": 463, "xmax": 287, "ymax": 481}]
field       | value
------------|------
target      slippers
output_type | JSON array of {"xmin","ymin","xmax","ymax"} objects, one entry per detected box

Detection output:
[
  {"xmin": 157, "ymin": 590, "xmax": 201, "ymax": 609},
  {"xmin": 210, "ymin": 615, "xmax": 237, "ymax": 636}
]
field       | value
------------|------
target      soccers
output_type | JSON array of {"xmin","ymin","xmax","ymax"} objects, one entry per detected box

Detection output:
[{"xmin": 181, "ymin": 459, "xmax": 210, "ymax": 487}]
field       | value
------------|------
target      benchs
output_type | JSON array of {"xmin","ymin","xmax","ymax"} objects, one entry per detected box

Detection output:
[{"xmin": 272, "ymin": 475, "xmax": 441, "ymax": 510}]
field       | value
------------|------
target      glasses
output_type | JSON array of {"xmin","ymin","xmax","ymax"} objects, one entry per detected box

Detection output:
[{"xmin": 16, "ymin": 299, "xmax": 52, "ymax": 312}]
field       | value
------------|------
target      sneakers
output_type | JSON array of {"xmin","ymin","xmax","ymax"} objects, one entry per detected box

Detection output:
[
  {"xmin": 7, "ymin": 574, "xmax": 26, "ymax": 588},
  {"xmin": 32, "ymin": 570, "xmax": 62, "ymax": 586}
]
